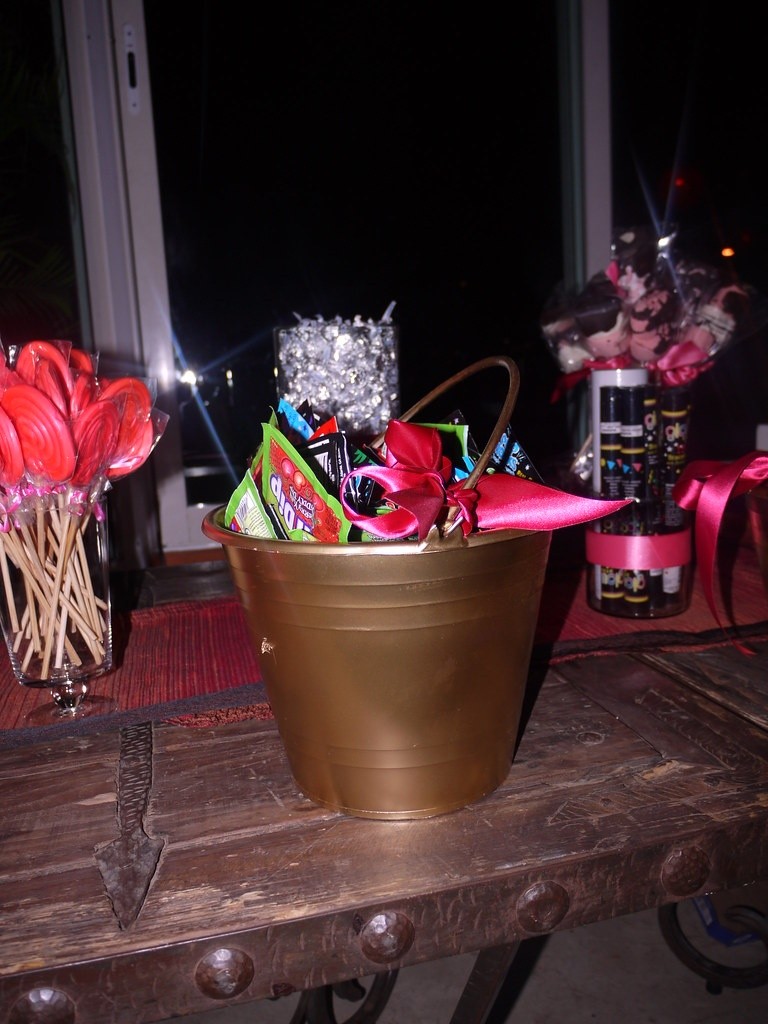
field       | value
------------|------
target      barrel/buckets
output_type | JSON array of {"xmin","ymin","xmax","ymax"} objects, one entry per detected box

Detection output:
[{"xmin": 201, "ymin": 356, "xmax": 554, "ymax": 822}]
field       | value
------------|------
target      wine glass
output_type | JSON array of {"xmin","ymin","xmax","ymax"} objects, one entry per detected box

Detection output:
[{"xmin": 0, "ymin": 496, "xmax": 119, "ymax": 725}]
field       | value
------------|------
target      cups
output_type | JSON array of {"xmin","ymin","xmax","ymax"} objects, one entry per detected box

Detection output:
[{"xmin": 580, "ymin": 482, "xmax": 698, "ymax": 618}]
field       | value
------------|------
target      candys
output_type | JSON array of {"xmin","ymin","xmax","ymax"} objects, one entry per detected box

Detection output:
[{"xmin": 1, "ymin": 341, "xmax": 153, "ymax": 490}]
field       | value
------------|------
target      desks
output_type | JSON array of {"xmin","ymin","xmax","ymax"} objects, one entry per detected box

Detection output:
[{"xmin": 0, "ymin": 538, "xmax": 768, "ymax": 1024}]
